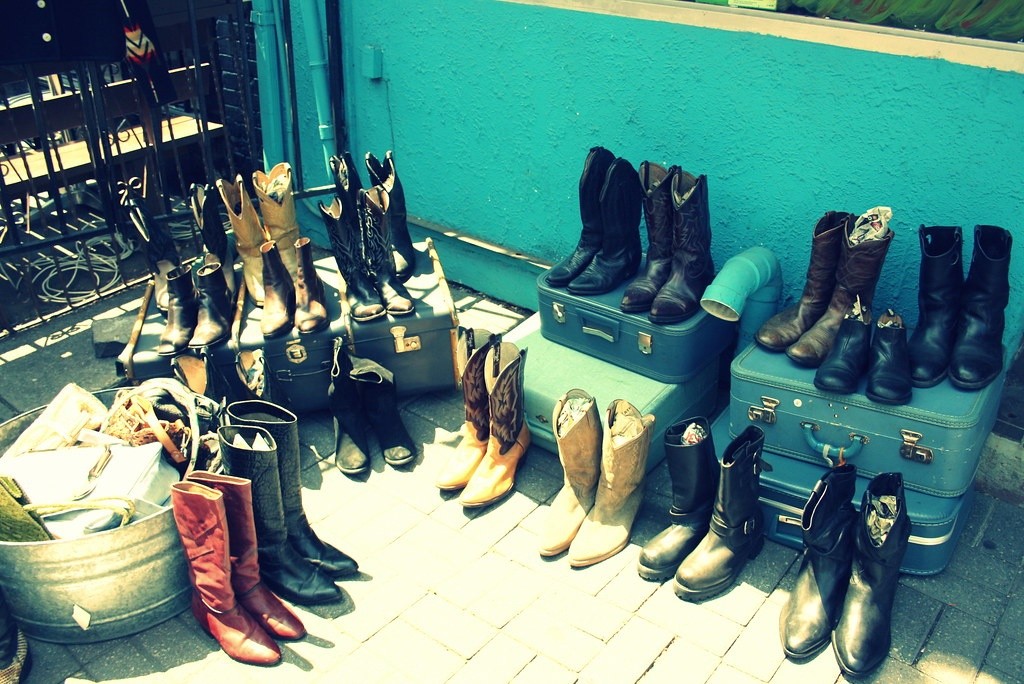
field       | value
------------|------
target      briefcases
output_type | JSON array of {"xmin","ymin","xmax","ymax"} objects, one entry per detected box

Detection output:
[
  {"xmin": 116, "ymin": 240, "xmax": 459, "ymax": 412},
  {"xmin": 514, "ymin": 252, "xmax": 1013, "ymax": 577}
]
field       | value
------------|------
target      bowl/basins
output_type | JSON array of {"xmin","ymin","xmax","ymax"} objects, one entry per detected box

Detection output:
[{"xmin": 0, "ymin": 387, "xmax": 230, "ymax": 644}]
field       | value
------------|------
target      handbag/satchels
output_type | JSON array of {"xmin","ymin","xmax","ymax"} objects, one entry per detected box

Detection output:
[{"xmin": 0, "ymin": 380, "xmax": 229, "ymax": 545}]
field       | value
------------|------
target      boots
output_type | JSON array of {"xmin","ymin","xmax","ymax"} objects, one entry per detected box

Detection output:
[
  {"xmin": 544, "ymin": 145, "xmax": 1014, "ymax": 406},
  {"xmin": 433, "ymin": 326, "xmax": 532, "ymax": 509},
  {"xmin": 122, "ymin": 149, "xmax": 416, "ymax": 358},
  {"xmin": 636, "ymin": 416, "xmax": 766, "ymax": 603},
  {"xmin": 172, "ymin": 345, "xmax": 272, "ymax": 406},
  {"xmin": 328, "ymin": 336, "xmax": 418, "ymax": 475},
  {"xmin": 172, "ymin": 469, "xmax": 308, "ymax": 668},
  {"xmin": 536, "ymin": 388, "xmax": 655, "ymax": 567},
  {"xmin": 217, "ymin": 399, "xmax": 359, "ymax": 607},
  {"xmin": 778, "ymin": 466, "xmax": 912, "ymax": 677}
]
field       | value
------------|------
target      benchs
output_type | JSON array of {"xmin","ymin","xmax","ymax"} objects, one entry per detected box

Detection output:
[{"xmin": 0, "ymin": 62, "xmax": 224, "ymax": 233}]
